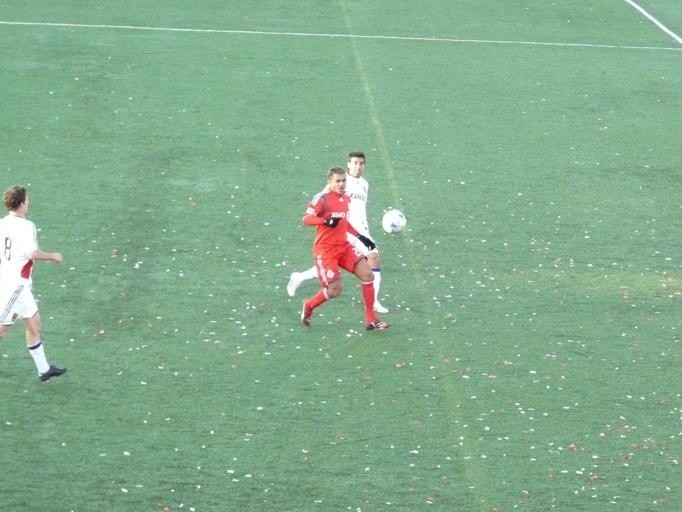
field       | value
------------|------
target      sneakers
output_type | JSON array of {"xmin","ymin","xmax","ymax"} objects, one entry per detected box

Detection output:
[
  {"xmin": 39, "ymin": 365, "xmax": 67, "ymax": 381},
  {"xmin": 373, "ymin": 302, "xmax": 389, "ymax": 313},
  {"xmin": 302, "ymin": 302, "xmax": 313, "ymax": 325},
  {"xmin": 287, "ymin": 272, "xmax": 301, "ymax": 296},
  {"xmin": 366, "ymin": 319, "xmax": 390, "ymax": 330}
]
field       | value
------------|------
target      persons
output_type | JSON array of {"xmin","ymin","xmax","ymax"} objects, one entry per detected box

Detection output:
[
  {"xmin": 0, "ymin": 185, "xmax": 68, "ymax": 382},
  {"xmin": 301, "ymin": 167, "xmax": 391, "ymax": 331},
  {"xmin": 287, "ymin": 152, "xmax": 389, "ymax": 314}
]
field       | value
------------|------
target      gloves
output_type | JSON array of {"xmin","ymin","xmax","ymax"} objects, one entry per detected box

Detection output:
[
  {"xmin": 359, "ymin": 236, "xmax": 375, "ymax": 250},
  {"xmin": 324, "ymin": 218, "xmax": 341, "ymax": 228}
]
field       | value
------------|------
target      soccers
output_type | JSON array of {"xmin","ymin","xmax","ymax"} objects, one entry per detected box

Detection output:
[{"xmin": 382, "ymin": 209, "xmax": 406, "ymax": 234}]
